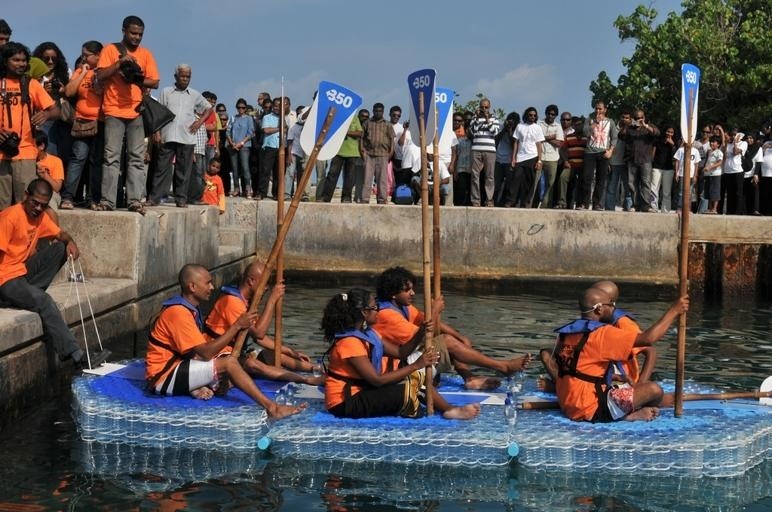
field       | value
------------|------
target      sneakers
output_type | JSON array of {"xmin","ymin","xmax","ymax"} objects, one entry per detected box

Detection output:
[{"xmin": 74, "ymin": 348, "xmax": 112, "ymax": 374}]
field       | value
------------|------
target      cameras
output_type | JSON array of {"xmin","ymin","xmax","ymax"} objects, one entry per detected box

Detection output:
[
  {"xmin": 119, "ymin": 59, "xmax": 145, "ymax": 84},
  {"xmin": 631, "ymin": 119, "xmax": 641, "ymax": 126},
  {"xmin": 0, "ymin": 131, "xmax": 21, "ymax": 159},
  {"xmin": 44, "ymin": 76, "xmax": 64, "ymax": 101},
  {"xmin": 480, "ymin": 110, "xmax": 484, "ymax": 116}
]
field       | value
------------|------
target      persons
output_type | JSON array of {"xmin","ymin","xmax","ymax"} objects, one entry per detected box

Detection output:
[
  {"xmin": 200, "ymin": 89, "xmax": 772, "ymax": 213},
  {"xmin": 536, "ymin": 280, "xmax": 656, "ymax": 394},
  {"xmin": 318, "ymin": 287, "xmax": 481, "ymax": 420},
  {"xmin": 368, "ymin": 265, "xmax": 532, "ymax": 391},
  {"xmin": 554, "ymin": 286, "xmax": 690, "ymax": 423},
  {"xmin": 0, "ymin": 16, "xmax": 215, "ymax": 213},
  {"xmin": 0, "ymin": 178, "xmax": 112, "ymax": 373},
  {"xmin": 145, "ymin": 263, "xmax": 309, "ymax": 420},
  {"xmin": 193, "ymin": 262, "xmax": 328, "ymax": 386}
]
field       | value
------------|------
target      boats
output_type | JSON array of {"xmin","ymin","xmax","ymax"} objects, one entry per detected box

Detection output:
[{"xmin": 72, "ymin": 329, "xmax": 768, "ymax": 479}]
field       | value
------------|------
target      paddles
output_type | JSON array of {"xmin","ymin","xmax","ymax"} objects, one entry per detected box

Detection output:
[
  {"xmin": 229, "ymin": 80, "xmax": 362, "ymax": 356},
  {"xmin": 406, "ymin": 68, "xmax": 437, "ymax": 411},
  {"xmin": 427, "ymin": 87, "xmax": 453, "ymax": 336},
  {"xmin": 674, "ymin": 62, "xmax": 702, "ymax": 415},
  {"xmin": 522, "ymin": 377, "xmax": 771, "ymax": 409},
  {"xmin": 275, "ymin": 76, "xmax": 285, "ymax": 366}
]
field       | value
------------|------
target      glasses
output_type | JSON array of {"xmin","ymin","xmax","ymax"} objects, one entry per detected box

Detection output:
[
  {"xmin": 597, "ymin": 301, "xmax": 617, "ymax": 309},
  {"xmin": 367, "ymin": 303, "xmax": 378, "ymax": 314}
]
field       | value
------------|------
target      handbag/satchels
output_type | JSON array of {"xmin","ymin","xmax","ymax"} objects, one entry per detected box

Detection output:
[{"xmin": 135, "ymin": 93, "xmax": 177, "ymax": 136}]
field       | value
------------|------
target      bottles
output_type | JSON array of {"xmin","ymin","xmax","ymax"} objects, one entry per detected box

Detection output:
[
  {"xmin": 314, "ymin": 359, "xmax": 322, "ymax": 377},
  {"xmin": 276, "ymin": 389, "xmax": 287, "ymax": 405},
  {"xmin": 508, "ymin": 376, "xmax": 517, "ymax": 391},
  {"xmin": 506, "ymin": 392, "xmax": 516, "ymax": 410},
  {"xmin": 287, "ymin": 384, "xmax": 294, "ymax": 403},
  {"xmin": 504, "ymin": 399, "xmax": 514, "ymax": 428}
]
